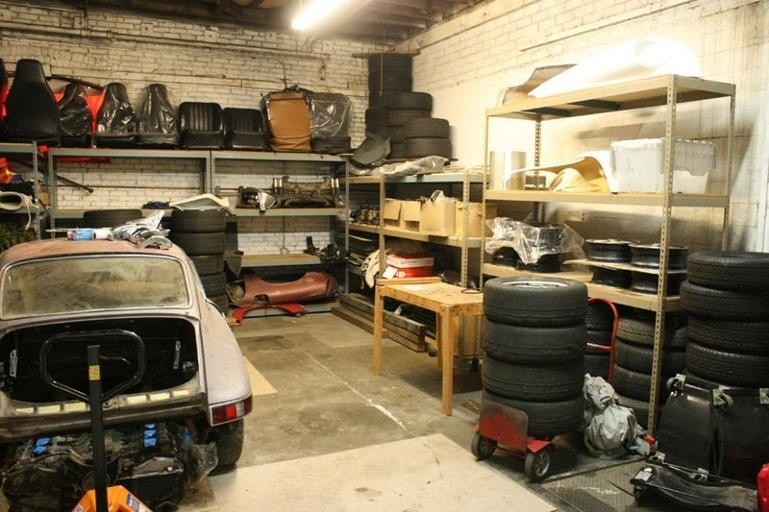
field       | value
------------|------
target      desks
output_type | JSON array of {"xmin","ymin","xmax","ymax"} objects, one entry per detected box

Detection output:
[{"xmin": 371, "ymin": 275, "xmax": 487, "ymax": 418}]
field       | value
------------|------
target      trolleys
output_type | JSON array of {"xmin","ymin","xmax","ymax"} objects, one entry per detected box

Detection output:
[{"xmin": 470, "ymin": 296, "xmax": 620, "ymax": 482}]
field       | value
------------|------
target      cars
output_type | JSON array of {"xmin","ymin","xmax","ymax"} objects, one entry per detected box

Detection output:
[{"xmin": 0, "ymin": 240, "xmax": 253, "ymax": 472}]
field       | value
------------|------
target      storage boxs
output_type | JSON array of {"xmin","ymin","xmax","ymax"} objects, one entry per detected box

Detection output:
[
  {"xmin": 384, "ymin": 194, "xmax": 499, "ymax": 238},
  {"xmin": 386, "ymin": 252, "xmax": 436, "ymax": 280}
]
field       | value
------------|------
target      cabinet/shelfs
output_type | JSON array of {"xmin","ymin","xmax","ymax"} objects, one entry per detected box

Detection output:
[
  {"xmin": 481, "ymin": 73, "xmax": 738, "ymax": 447},
  {"xmin": 47, "ymin": 149, "xmax": 347, "ymax": 317},
  {"xmin": 333, "ymin": 171, "xmax": 488, "ymax": 354},
  {"xmin": 0, "ymin": 142, "xmax": 40, "ymax": 243}
]
